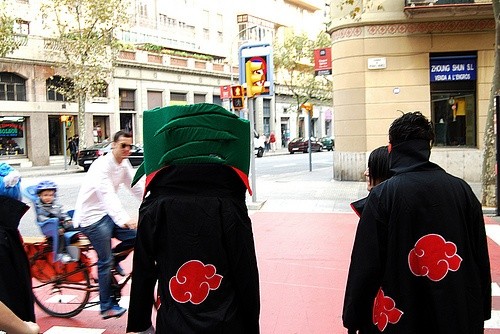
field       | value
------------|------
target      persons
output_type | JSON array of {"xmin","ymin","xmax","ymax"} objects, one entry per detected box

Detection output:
[
  {"xmin": 342, "ymin": 110, "xmax": 492, "ymax": 334},
  {"xmin": 34, "ymin": 181, "xmax": 75, "ymax": 264},
  {"xmin": 126, "ymin": 101, "xmax": 261, "ymax": 333},
  {"xmin": 261, "ymin": 131, "xmax": 276, "ymax": 152},
  {"xmin": 0, "ymin": 163, "xmax": 37, "ymax": 323},
  {"xmin": 66, "ymin": 135, "xmax": 80, "ymax": 165},
  {"xmin": 72, "ymin": 131, "xmax": 144, "ymax": 319},
  {"xmin": 0, "ymin": 137, "xmax": 20, "ymax": 155},
  {"xmin": 97, "ymin": 125, "xmax": 102, "ymax": 143},
  {"xmin": 0, "ymin": 301, "xmax": 40, "ymax": 334},
  {"xmin": 102, "ymin": 136, "xmax": 109, "ymax": 143}
]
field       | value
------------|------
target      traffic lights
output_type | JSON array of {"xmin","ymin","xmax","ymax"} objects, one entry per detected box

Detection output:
[
  {"xmin": 64, "ymin": 115, "xmax": 74, "ymax": 127},
  {"xmin": 246, "ymin": 61, "xmax": 266, "ymax": 98},
  {"xmin": 231, "ymin": 85, "xmax": 244, "ymax": 109}
]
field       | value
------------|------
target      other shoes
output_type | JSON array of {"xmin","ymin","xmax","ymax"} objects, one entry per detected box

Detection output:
[
  {"xmin": 100, "ymin": 305, "xmax": 126, "ymax": 318},
  {"xmin": 56, "ymin": 252, "xmax": 71, "ymax": 264},
  {"xmin": 113, "ymin": 263, "xmax": 125, "ymax": 276}
]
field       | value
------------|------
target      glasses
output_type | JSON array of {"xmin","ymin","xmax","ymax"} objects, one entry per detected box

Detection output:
[{"xmin": 116, "ymin": 142, "xmax": 134, "ymax": 150}]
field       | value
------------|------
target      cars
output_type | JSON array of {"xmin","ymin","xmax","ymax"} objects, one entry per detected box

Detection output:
[
  {"xmin": 253, "ymin": 129, "xmax": 265, "ymax": 157},
  {"xmin": 77, "ymin": 141, "xmax": 144, "ymax": 171},
  {"xmin": 288, "ymin": 136, "xmax": 335, "ymax": 154}
]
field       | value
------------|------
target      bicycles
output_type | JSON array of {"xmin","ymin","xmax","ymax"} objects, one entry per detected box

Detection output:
[{"xmin": 20, "ymin": 234, "xmax": 161, "ymax": 319}]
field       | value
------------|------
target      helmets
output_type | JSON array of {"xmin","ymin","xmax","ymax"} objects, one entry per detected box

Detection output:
[{"xmin": 35, "ymin": 180, "xmax": 58, "ymax": 194}]
